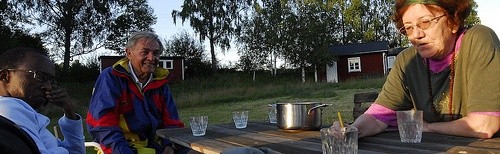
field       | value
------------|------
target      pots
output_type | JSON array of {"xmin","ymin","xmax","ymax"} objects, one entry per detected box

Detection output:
[{"xmin": 268, "ymin": 101, "xmax": 333, "ymax": 129}]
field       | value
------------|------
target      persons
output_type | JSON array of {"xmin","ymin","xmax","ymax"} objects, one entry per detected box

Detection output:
[
  {"xmin": 329, "ymin": 0, "xmax": 500, "ymax": 139},
  {"xmin": 0, "ymin": 47, "xmax": 86, "ymax": 154},
  {"xmin": 85, "ymin": 31, "xmax": 185, "ymax": 154}
]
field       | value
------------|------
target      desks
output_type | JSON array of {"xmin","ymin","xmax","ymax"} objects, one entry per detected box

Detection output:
[{"xmin": 156, "ymin": 120, "xmax": 500, "ymax": 154}]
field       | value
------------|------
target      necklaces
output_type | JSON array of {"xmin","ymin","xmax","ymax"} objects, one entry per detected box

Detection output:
[{"xmin": 423, "ymin": 52, "xmax": 456, "ymax": 122}]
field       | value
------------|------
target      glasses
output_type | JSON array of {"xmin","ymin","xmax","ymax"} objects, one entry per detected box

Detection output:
[
  {"xmin": 400, "ymin": 14, "xmax": 445, "ymax": 36},
  {"xmin": 0, "ymin": 68, "xmax": 56, "ymax": 85}
]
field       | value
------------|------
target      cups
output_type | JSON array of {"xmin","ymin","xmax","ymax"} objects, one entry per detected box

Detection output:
[
  {"xmin": 319, "ymin": 126, "xmax": 358, "ymax": 154},
  {"xmin": 232, "ymin": 110, "xmax": 249, "ymax": 129},
  {"xmin": 268, "ymin": 104, "xmax": 278, "ymax": 123},
  {"xmin": 187, "ymin": 116, "xmax": 209, "ymax": 137},
  {"xmin": 396, "ymin": 110, "xmax": 423, "ymax": 143}
]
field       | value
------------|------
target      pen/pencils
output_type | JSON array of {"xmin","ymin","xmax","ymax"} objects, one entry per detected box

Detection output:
[{"xmin": 338, "ymin": 111, "xmax": 351, "ymax": 153}]
